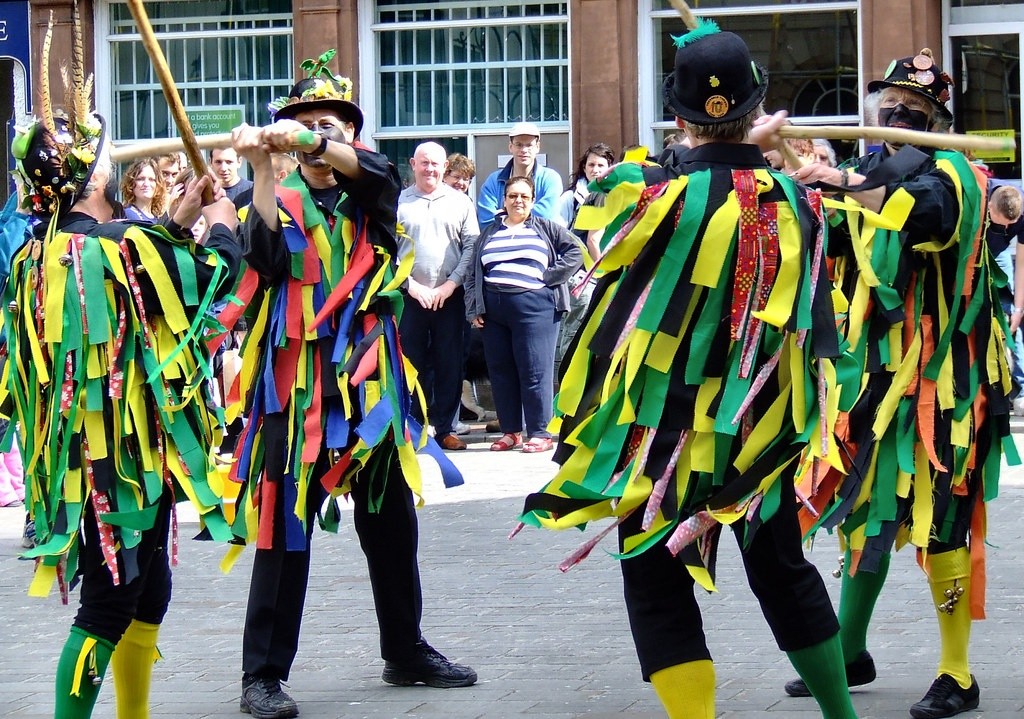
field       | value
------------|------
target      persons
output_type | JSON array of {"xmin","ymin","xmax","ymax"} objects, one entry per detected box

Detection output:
[
  {"xmin": 0, "ymin": 116, "xmax": 1024, "ymax": 508},
  {"xmin": 784, "ymin": 47, "xmax": 1024, "ymax": 719},
  {"xmin": 510, "ymin": 20, "xmax": 861, "ymax": 719},
  {"xmin": 0, "ymin": 113, "xmax": 240, "ymax": 719},
  {"xmin": 231, "ymin": 47, "xmax": 478, "ymax": 719}
]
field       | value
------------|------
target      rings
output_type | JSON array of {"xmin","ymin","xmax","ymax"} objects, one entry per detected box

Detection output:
[{"xmin": 472, "ymin": 323, "xmax": 476, "ymax": 326}]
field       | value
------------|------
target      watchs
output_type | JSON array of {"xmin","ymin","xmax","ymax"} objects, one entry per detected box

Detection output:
[{"xmin": 839, "ymin": 168, "xmax": 850, "ymax": 196}]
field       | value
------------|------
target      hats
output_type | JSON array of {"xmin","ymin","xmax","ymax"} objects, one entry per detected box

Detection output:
[
  {"xmin": 867, "ymin": 48, "xmax": 955, "ymax": 123},
  {"xmin": 509, "ymin": 122, "xmax": 541, "ymax": 142},
  {"xmin": 9, "ymin": 7, "xmax": 106, "ymax": 221},
  {"xmin": 267, "ymin": 49, "xmax": 364, "ymax": 140},
  {"xmin": 663, "ymin": 18, "xmax": 770, "ymax": 126}
]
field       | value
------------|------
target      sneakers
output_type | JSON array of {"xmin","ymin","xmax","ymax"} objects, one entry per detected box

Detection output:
[
  {"xmin": 451, "ymin": 421, "xmax": 470, "ymax": 434},
  {"xmin": 784, "ymin": 649, "xmax": 877, "ymax": 697},
  {"xmin": 443, "ymin": 434, "xmax": 467, "ymax": 450},
  {"xmin": 908, "ymin": 673, "xmax": 981, "ymax": 719},
  {"xmin": 486, "ymin": 420, "xmax": 501, "ymax": 433},
  {"xmin": 239, "ymin": 675, "xmax": 300, "ymax": 719},
  {"xmin": 381, "ymin": 636, "xmax": 479, "ymax": 689}
]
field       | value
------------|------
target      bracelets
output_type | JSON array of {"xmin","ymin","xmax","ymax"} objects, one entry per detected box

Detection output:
[
  {"xmin": 1014, "ymin": 307, "xmax": 1024, "ymax": 315},
  {"xmin": 303, "ymin": 132, "xmax": 327, "ymax": 158}
]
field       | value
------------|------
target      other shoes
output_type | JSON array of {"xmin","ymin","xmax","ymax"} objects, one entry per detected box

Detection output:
[{"xmin": 476, "ymin": 413, "xmax": 487, "ymax": 422}]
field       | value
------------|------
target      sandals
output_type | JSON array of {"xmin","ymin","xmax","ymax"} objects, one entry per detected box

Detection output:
[
  {"xmin": 521, "ymin": 436, "xmax": 555, "ymax": 453},
  {"xmin": 490, "ymin": 433, "xmax": 523, "ymax": 452}
]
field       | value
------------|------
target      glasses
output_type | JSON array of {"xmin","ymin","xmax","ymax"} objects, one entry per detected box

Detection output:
[
  {"xmin": 511, "ymin": 142, "xmax": 535, "ymax": 149},
  {"xmin": 506, "ymin": 194, "xmax": 531, "ymax": 202},
  {"xmin": 880, "ymin": 94, "xmax": 930, "ymax": 111}
]
